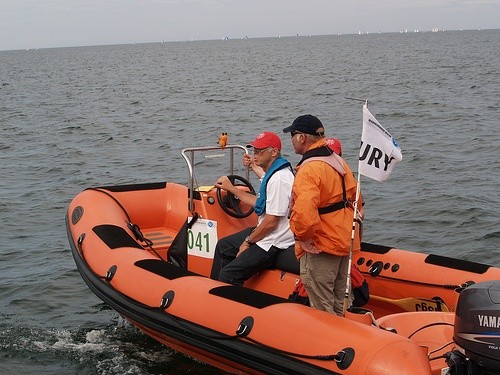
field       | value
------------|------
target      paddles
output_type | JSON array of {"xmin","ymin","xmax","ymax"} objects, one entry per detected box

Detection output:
[{"xmin": 369, "ymin": 294, "xmax": 449, "ymax": 312}]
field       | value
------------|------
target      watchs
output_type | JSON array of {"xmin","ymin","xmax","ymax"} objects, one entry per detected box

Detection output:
[{"xmin": 244, "ymin": 236, "xmax": 253, "ymax": 245}]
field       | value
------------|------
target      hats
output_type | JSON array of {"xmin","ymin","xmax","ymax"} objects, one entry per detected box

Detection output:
[
  {"xmin": 283, "ymin": 114, "xmax": 324, "ymax": 136},
  {"xmin": 246, "ymin": 132, "xmax": 281, "ymax": 150},
  {"xmin": 326, "ymin": 138, "xmax": 342, "ymax": 155}
]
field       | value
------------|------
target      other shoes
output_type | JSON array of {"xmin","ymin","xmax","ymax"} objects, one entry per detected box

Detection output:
[{"xmin": 352, "ymin": 278, "xmax": 368, "ymax": 306}]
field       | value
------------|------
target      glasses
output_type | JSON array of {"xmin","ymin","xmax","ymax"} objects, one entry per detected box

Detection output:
[
  {"xmin": 291, "ymin": 131, "xmax": 309, "ymax": 143},
  {"xmin": 253, "ymin": 148, "xmax": 271, "ymax": 155}
]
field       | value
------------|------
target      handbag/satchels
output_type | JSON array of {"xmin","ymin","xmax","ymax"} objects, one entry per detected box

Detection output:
[{"xmin": 168, "ymin": 212, "xmax": 198, "ymax": 270}]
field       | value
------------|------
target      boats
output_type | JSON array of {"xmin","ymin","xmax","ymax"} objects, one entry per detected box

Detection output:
[{"xmin": 64, "ymin": 182, "xmax": 500, "ymax": 375}]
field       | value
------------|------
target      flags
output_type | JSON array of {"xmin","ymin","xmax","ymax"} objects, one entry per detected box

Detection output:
[{"xmin": 356, "ymin": 102, "xmax": 403, "ymax": 184}]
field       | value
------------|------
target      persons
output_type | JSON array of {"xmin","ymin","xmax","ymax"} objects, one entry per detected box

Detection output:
[
  {"xmin": 210, "ymin": 130, "xmax": 297, "ymax": 286},
  {"xmin": 215, "ymin": 132, "xmax": 228, "ymax": 151},
  {"xmin": 283, "ymin": 114, "xmax": 369, "ymax": 316}
]
